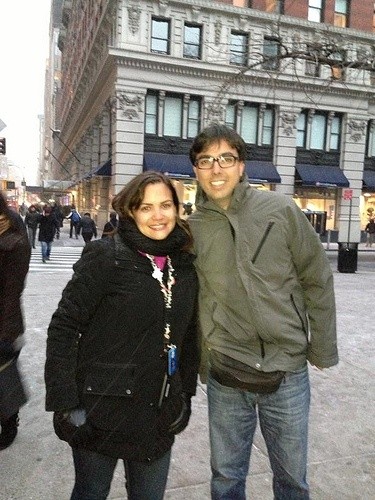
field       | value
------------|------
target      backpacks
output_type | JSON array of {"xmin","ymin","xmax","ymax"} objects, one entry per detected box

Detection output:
[{"xmin": 72, "ymin": 211, "xmax": 80, "ymax": 222}]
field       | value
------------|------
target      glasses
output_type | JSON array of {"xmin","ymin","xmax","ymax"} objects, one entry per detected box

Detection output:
[{"xmin": 195, "ymin": 157, "xmax": 242, "ymax": 169}]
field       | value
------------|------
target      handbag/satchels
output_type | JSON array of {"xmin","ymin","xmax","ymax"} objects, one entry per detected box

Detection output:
[{"xmin": 210, "ymin": 349, "xmax": 283, "ymax": 393}]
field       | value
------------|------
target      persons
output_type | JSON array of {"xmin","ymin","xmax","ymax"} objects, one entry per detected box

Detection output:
[
  {"xmin": 20, "ymin": 201, "xmax": 65, "ymax": 264},
  {"xmin": 184, "ymin": 122, "xmax": 340, "ymax": 500},
  {"xmin": 0, "ymin": 189, "xmax": 31, "ymax": 450},
  {"xmin": 77, "ymin": 213, "xmax": 97, "ymax": 244},
  {"xmin": 101, "ymin": 212, "xmax": 118, "ymax": 240},
  {"xmin": 45, "ymin": 170, "xmax": 201, "ymax": 500},
  {"xmin": 364, "ymin": 219, "xmax": 375, "ymax": 247},
  {"xmin": 65, "ymin": 204, "xmax": 81, "ymax": 240}
]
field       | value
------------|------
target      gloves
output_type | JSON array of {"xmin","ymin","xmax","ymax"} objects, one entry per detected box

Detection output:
[
  {"xmin": 169, "ymin": 392, "xmax": 191, "ymax": 435},
  {"xmin": 53, "ymin": 406, "xmax": 87, "ymax": 448}
]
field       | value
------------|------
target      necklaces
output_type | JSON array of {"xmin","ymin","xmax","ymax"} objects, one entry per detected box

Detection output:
[{"xmin": 143, "ymin": 252, "xmax": 174, "ymax": 352}]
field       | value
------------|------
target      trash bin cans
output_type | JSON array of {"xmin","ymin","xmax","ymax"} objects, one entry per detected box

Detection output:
[{"xmin": 337, "ymin": 242, "xmax": 359, "ymax": 273}]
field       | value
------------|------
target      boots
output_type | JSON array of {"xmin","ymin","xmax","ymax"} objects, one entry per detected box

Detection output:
[{"xmin": 0, "ymin": 409, "xmax": 20, "ymax": 450}]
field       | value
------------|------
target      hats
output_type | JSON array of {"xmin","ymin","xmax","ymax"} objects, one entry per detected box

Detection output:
[
  {"xmin": 110, "ymin": 212, "xmax": 116, "ymax": 217},
  {"xmin": 45, "ymin": 208, "xmax": 51, "ymax": 213}
]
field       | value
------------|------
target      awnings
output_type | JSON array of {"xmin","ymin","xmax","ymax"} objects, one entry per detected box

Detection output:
[
  {"xmin": 143, "ymin": 151, "xmax": 282, "ymax": 184},
  {"xmin": 362, "ymin": 171, "xmax": 375, "ymax": 193},
  {"xmin": 295, "ymin": 164, "xmax": 349, "ymax": 188},
  {"xmin": 93, "ymin": 160, "xmax": 111, "ymax": 177}
]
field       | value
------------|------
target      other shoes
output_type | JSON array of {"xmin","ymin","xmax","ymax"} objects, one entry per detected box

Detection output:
[
  {"xmin": 46, "ymin": 257, "xmax": 49, "ymax": 260},
  {"xmin": 43, "ymin": 257, "xmax": 46, "ymax": 263}
]
